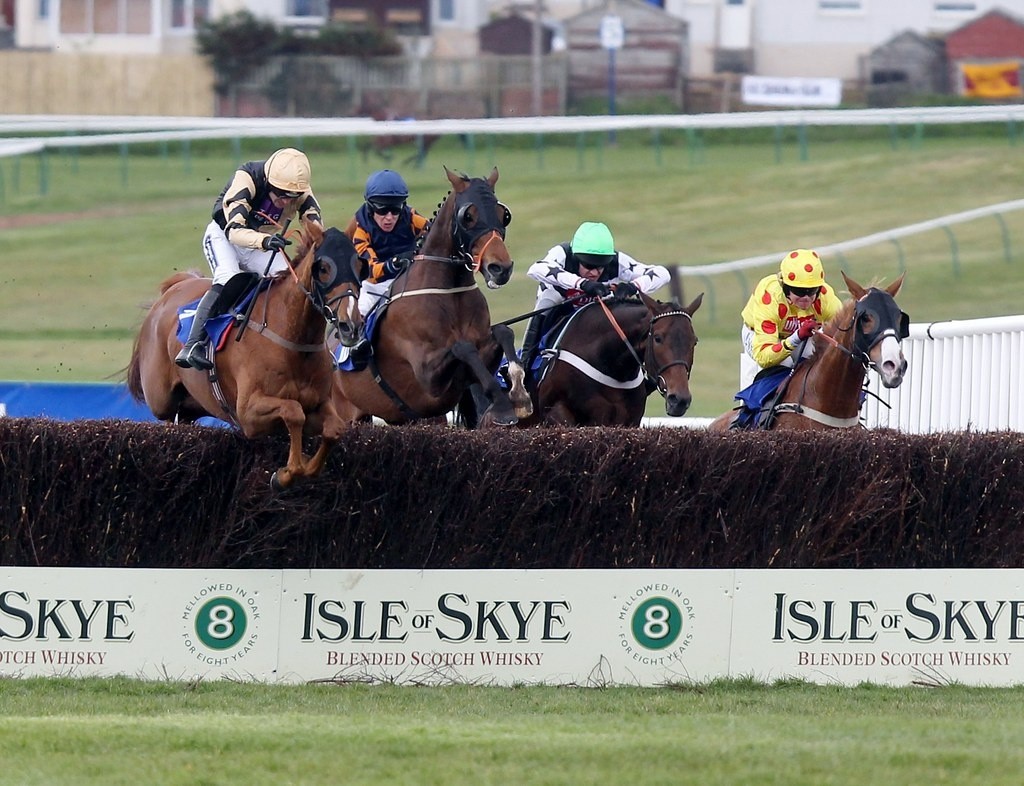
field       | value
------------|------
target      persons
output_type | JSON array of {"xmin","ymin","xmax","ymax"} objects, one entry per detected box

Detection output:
[
  {"xmin": 502, "ymin": 221, "xmax": 672, "ymax": 387},
  {"xmin": 344, "ymin": 169, "xmax": 432, "ymax": 368},
  {"xmin": 175, "ymin": 148, "xmax": 324, "ymax": 370},
  {"xmin": 741, "ymin": 249, "xmax": 843, "ymax": 378}
]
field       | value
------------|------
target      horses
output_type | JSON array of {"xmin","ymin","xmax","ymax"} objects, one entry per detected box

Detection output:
[
  {"xmin": 708, "ymin": 271, "xmax": 910, "ymax": 433},
  {"xmin": 102, "ymin": 217, "xmax": 369, "ymax": 493},
  {"xmin": 327, "ymin": 165, "xmax": 533, "ymax": 430},
  {"xmin": 450, "ymin": 291, "xmax": 707, "ymax": 429}
]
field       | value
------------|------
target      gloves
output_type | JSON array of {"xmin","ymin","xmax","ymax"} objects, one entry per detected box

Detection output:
[
  {"xmin": 798, "ymin": 320, "xmax": 822, "ymax": 340},
  {"xmin": 262, "ymin": 233, "xmax": 293, "ymax": 253},
  {"xmin": 613, "ymin": 280, "xmax": 640, "ymax": 302},
  {"xmin": 580, "ymin": 279, "xmax": 607, "ymax": 299},
  {"xmin": 391, "ymin": 253, "xmax": 413, "ymax": 270}
]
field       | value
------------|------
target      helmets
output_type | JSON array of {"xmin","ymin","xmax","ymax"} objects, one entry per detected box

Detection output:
[
  {"xmin": 570, "ymin": 222, "xmax": 617, "ymax": 267},
  {"xmin": 265, "ymin": 147, "xmax": 311, "ymax": 190},
  {"xmin": 363, "ymin": 170, "xmax": 409, "ymax": 207},
  {"xmin": 781, "ymin": 248, "xmax": 825, "ymax": 288}
]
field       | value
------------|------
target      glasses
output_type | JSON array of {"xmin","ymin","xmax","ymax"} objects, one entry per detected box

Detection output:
[
  {"xmin": 577, "ymin": 260, "xmax": 602, "ymax": 270},
  {"xmin": 270, "ymin": 187, "xmax": 304, "ymax": 198},
  {"xmin": 366, "ymin": 199, "xmax": 402, "ymax": 216},
  {"xmin": 786, "ymin": 286, "xmax": 819, "ymax": 297}
]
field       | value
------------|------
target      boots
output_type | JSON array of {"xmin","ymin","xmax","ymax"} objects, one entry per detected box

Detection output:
[{"xmin": 172, "ymin": 290, "xmax": 220, "ymax": 371}]
